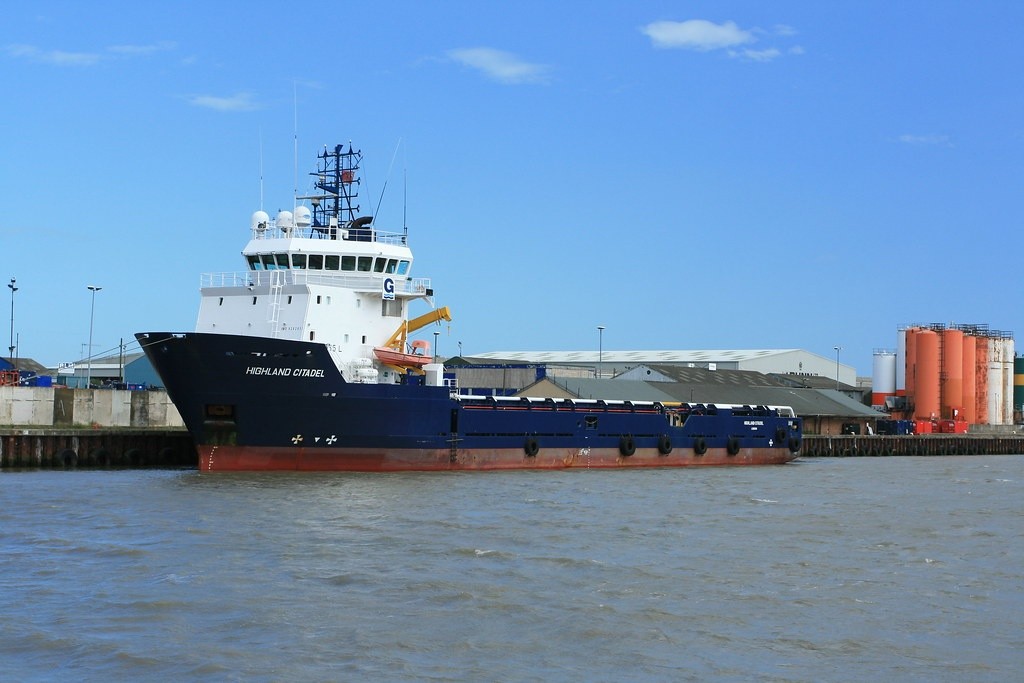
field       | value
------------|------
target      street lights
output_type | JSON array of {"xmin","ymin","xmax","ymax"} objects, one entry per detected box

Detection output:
[
  {"xmin": 87, "ymin": 286, "xmax": 102, "ymax": 389},
  {"xmin": 8, "ymin": 277, "xmax": 18, "ymax": 363},
  {"xmin": 434, "ymin": 332, "xmax": 440, "ymax": 362},
  {"xmin": 597, "ymin": 326, "xmax": 605, "ymax": 378},
  {"xmin": 834, "ymin": 347, "xmax": 843, "ymax": 390}
]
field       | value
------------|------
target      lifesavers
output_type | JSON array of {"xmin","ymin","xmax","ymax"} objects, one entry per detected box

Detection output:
[
  {"xmin": 849, "ymin": 444, "xmax": 988, "ymax": 456},
  {"xmin": 823, "ymin": 447, "xmax": 833, "ymax": 457},
  {"xmin": 619, "ymin": 436, "xmax": 636, "ymax": 457},
  {"xmin": 53, "ymin": 448, "xmax": 79, "ymax": 469},
  {"xmin": 836, "ymin": 445, "xmax": 845, "ymax": 457},
  {"xmin": 775, "ymin": 428, "xmax": 786, "ymax": 443},
  {"xmin": 417, "ymin": 285, "xmax": 424, "ymax": 292},
  {"xmin": 89, "ymin": 448, "xmax": 113, "ymax": 468},
  {"xmin": 123, "ymin": 448, "xmax": 146, "ymax": 468},
  {"xmin": 789, "ymin": 436, "xmax": 801, "ymax": 452},
  {"xmin": 524, "ymin": 438, "xmax": 540, "ymax": 457},
  {"xmin": 658, "ymin": 437, "xmax": 673, "ymax": 455},
  {"xmin": 727, "ymin": 439, "xmax": 740, "ymax": 455},
  {"xmin": 694, "ymin": 437, "xmax": 708, "ymax": 454},
  {"xmin": 809, "ymin": 447, "xmax": 821, "ymax": 457}
]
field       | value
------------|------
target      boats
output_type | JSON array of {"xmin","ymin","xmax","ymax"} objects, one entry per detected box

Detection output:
[
  {"xmin": 373, "ymin": 346, "xmax": 433, "ymax": 366},
  {"xmin": 134, "ymin": 77, "xmax": 802, "ymax": 472}
]
